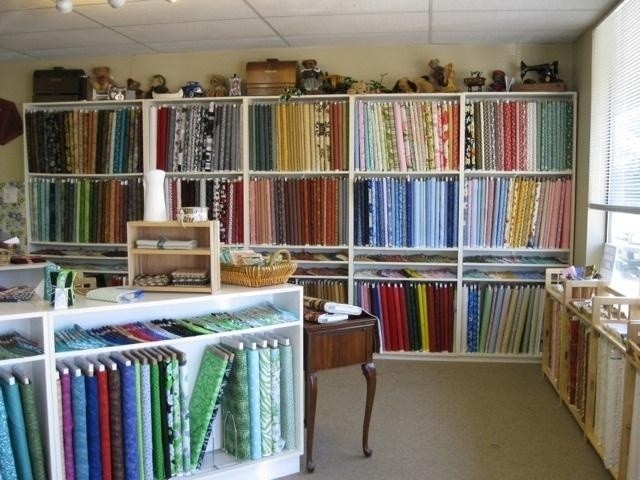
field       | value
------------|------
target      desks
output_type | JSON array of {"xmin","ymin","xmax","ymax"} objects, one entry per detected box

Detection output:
[{"xmin": 304, "ymin": 317, "xmax": 378, "ymax": 475}]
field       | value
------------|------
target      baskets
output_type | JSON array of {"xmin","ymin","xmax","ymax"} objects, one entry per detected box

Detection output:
[{"xmin": 221, "ymin": 249, "xmax": 298, "ymax": 287}]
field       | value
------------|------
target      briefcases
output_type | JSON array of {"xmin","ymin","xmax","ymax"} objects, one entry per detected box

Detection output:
[
  {"xmin": 33, "ymin": 67, "xmax": 86, "ymax": 102},
  {"xmin": 246, "ymin": 59, "xmax": 298, "ymax": 96}
]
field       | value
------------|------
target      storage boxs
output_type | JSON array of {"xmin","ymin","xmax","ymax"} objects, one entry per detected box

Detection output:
[{"xmin": 244, "ymin": 56, "xmax": 298, "ymax": 97}]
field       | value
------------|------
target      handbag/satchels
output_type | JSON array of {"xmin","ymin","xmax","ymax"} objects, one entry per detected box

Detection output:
[{"xmin": 87, "ymin": 287, "xmax": 143, "ymax": 303}]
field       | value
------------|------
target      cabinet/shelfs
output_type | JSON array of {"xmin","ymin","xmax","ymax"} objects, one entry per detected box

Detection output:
[
  {"xmin": 126, "ymin": 219, "xmax": 222, "ymax": 295},
  {"xmin": 543, "ymin": 267, "xmax": 640, "ymax": 480}
]
